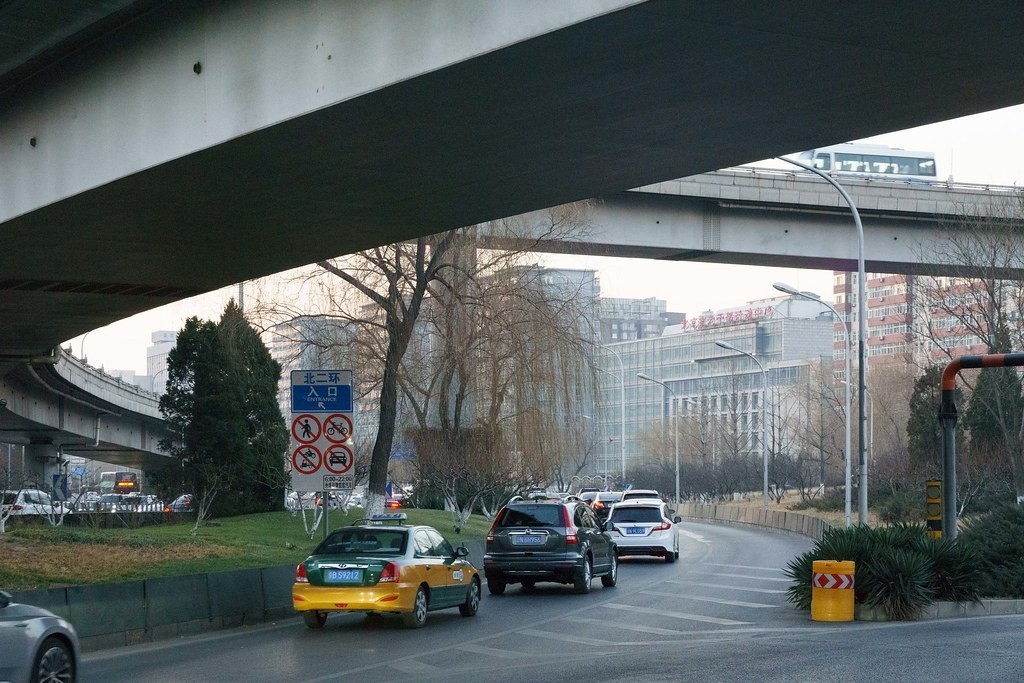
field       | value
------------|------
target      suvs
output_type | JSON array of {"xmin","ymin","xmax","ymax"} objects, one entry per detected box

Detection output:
[
  {"xmin": 484, "ymin": 496, "xmax": 618, "ymax": 594},
  {"xmin": 602, "ymin": 503, "xmax": 682, "ymax": 562},
  {"xmin": 620, "ymin": 489, "xmax": 662, "ymax": 504},
  {"xmin": 0, "ymin": 489, "xmax": 75, "ymax": 513}
]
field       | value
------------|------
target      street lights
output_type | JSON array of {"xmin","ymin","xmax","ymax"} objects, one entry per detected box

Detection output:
[
  {"xmin": 581, "ymin": 336, "xmax": 626, "ymax": 483},
  {"xmin": 715, "ymin": 340, "xmax": 768, "ymax": 508},
  {"xmin": 771, "ymin": 282, "xmax": 851, "ymax": 524},
  {"xmin": 636, "ymin": 373, "xmax": 680, "ymax": 503}
]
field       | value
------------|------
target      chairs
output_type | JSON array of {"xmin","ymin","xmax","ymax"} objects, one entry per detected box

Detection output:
[
  {"xmin": 363, "ymin": 536, "xmax": 377, "ymax": 541},
  {"xmin": 390, "ymin": 538, "xmax": 402, "ymax": 548}
]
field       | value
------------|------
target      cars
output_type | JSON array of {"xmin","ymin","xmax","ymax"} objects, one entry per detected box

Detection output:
[
  {"xmin": 384, "ymin": 494, "xmax": 411, "ymax": 509},
  {"xmin": 293, "ymin": 525, "xmax": 481, "ymax": 628},
  {"xmin": 577, "ymin": 488, "xmax": 601, "ymax": 502},
  {"xmin": 288, "ymin": 490, "xmax": 368, "ymax": 510},
  {"xmin": 591, "ymin": 492, "xmax": 623, "ymax": 521},
  {"xmin": 0, "ymin": 591, "xmax": 83, "ymax": 683},
  {"xmin": 166, "ymin": 494, "xmax": 193, "ymax": 512},
  {"xmin": 69, "ymin": 493, "xmax": 165, "ymax": 511}
]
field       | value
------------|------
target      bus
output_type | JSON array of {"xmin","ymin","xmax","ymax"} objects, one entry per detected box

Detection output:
[
  {"xmin": 796, "ymin": 141, "xmax": 936, "ymax": 183},
  {"xmin": 100, "ymin": 470, "xmax": 139, "ymax": 494}
]
